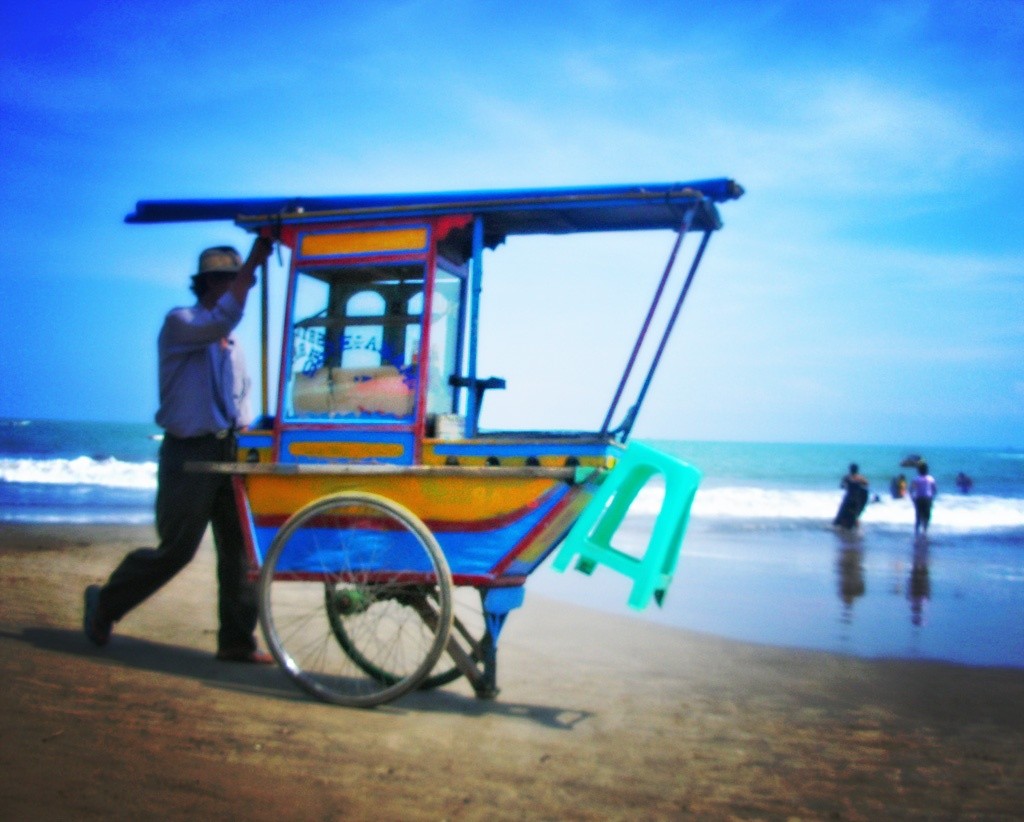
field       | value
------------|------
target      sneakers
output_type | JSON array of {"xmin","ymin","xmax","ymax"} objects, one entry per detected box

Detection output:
[
  {"xmin": 229, "ymin": 651, "xmax": 273, "ymax": 663},
  {"xmin": 84, "ymin": 586, "xmax": 112, "ymax": 645}
]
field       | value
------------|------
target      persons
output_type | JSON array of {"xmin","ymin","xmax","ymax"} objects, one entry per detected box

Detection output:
[
  {"xmin": 82, "ymin": 228, "xmax": 274, "ymax": 664},
  {"xmin": 954, "ymin": 472, "xmax": 973, "ymax": 494},
  {"xmin": 891, "ymin": 463, "xmax": 937, "ymax": 530},
  {"xmin": 832, "ymin": 464, "xmax": 868, "ymax": 530}
]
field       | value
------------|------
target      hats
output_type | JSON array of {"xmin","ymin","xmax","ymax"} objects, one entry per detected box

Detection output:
[{"xmin": 192, "ymin": 246, "xmax": 256, "ymax": 289}]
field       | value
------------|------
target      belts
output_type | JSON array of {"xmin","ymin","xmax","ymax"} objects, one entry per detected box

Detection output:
[{"xmin": 214, "ymin": 431, "xmax": 230, "ymax": 440}]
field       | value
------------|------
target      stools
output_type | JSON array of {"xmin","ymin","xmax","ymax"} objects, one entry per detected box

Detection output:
[{"xmin": 552, "ymin": 439, "xmax": 703, "ymax": 613}]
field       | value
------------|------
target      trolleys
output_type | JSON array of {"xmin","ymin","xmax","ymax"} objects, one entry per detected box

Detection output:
[{"xmin": 118, "ymin": 175, "xmax": 746, "ymax": 709}]
